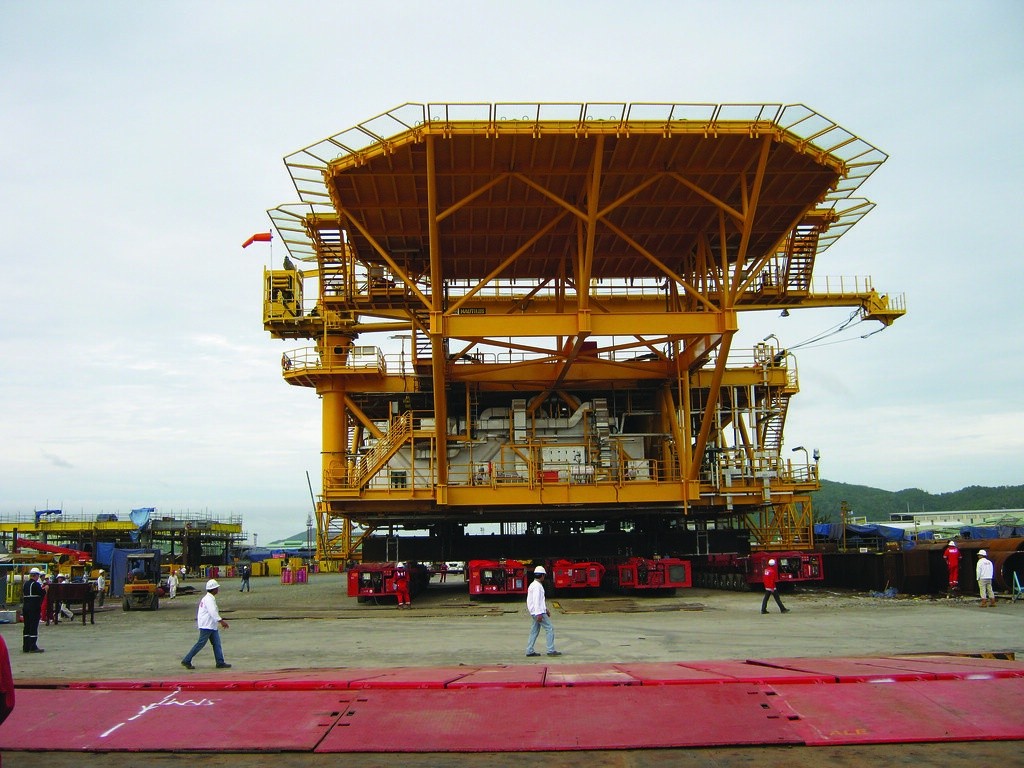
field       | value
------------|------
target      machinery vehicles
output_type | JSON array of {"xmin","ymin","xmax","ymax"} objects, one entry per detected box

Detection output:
[
  {"xmin": 122, "ymin": 553, "xmax": 161, "ymax": 611},
  {"xmin": 15, "ymin": 537, "xmax": 111, "ymax": 593}
]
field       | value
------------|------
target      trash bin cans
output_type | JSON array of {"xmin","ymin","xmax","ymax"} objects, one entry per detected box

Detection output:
[
  {"xmin": 390, "ymin": 471, "xmax": 406, "ymax": 488},
  {"xmin": 537, "ymin": 470, "xmax": 558, "ymax": 482}
]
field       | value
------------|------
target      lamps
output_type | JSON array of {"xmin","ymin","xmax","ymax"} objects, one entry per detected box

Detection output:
[
  {"xmin": 369, "ymin": 267, "xmax": 383, "ymax": 277},
  {"xmin": 734, "ymin": 270, "xmax": 747, "ymax": 280},
  {"xmin": 781, "ymin": 309, "xmax": 789, "ymax": 317}
]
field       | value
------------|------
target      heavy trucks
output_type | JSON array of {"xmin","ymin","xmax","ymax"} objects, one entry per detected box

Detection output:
[
  {"xmin": 467, "ymin": 560, "xmax": 529, "ymax": 599},
  {"xmin": 544, "ymin": 559, "xmax": 605, "ymax": 590},
  {"xmin": 605, "ymin": 557, "xmax": 692, "ymax": 596},
  {"xmin": 692, "ymin": 552, "xmax": 824, "ymax": 592},
  {"xmin": 347, "ymin": 561, "xmax": 430, "ymax": 605}
]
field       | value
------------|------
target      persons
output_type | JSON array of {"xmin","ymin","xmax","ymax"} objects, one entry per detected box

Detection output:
[
  {"xmin": 761, "ymin": 559, "xmax": 790, "ymax": 614},
  {"xmin": 39, "ymin": 570, "xmax": 73, "ymax": 626},
  {"xmin": 976, "ymin": 550, "xmax": 996, "ymax": 607},
  {"xmin": 132, "ymin": 561, "xmax": 144, "ymax": 575},
  {"xmin": 167, "ymin": 570, "xmax": 179, "ymax": 599},
  {"xmin": 393, "ymin": 562, "xmax": 411, "ymax": 609},
  {"xmin": 440, "ymin": 562, "xmax": 447, "ymax": 583},
  {"xmin": 239, "ymin": 566, "xmax": 249, "ymax": 592},
  {"xmin": 23, "ymin": 567, "xmax": 44, "ymax": 653},
  {"xmin": 943, "ymin": 540, "xmax": 962, "ymax": 590},
  {"xmin": 97, "ymin": 569, "xmax": 105, "ymax": 608},
  {"xmin": 525, "ymin": 566, "xmax": 562, "ymax": 656},
  {"xmin": 181, "ymin": 579, "xmax": 231, "ymax": 669}
]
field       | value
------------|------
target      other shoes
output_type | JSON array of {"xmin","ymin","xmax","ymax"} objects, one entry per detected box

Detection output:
[
  {"xmin": 24, "ymin": 648, "xmax": 44, "ymax": 653},
  {"xmin": 398, "ymin": 604, "xmax": 404, "ymax": 610},
  {"xmin": 408, "ymin": 604, "xmax": 411, "ymax": 610},
  {"xmin": 781, "ymin": 608, "xmax": 790, "ymax": 614},
  {"xmin": 180, "ymin": 660, "xmax": 195, "ymax": 670},
  {"xmin": 525, "ymin": 652, "xmax": 542, "ymax": 657},
  {"xmin": 216, "ymin": 663, "xmax": 232, "ymax": 668},
  {"xmin": 761, "ymin": 611, "xmax": 770, "ymax": 614},
  {"xmin": 547, "ymin": 651, "xmax": 563, "ymax": 656}
]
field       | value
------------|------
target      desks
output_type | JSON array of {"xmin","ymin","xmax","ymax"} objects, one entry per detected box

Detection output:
[{"xmin": 46, "ymin": 583, "xmax": 98, "ymax": 625}]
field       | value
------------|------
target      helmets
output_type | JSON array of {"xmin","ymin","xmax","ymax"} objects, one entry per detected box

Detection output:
[
  {"xmin": 768, "ymin": 559, "xmax": 775, "ymax": 566},
  {"xmin": 205, "ymin": 578, "xmax": 221, "ymax": 591},
  {"xmin": 243, "ymin": 566, "xmax": 247, "ymax": 569},
  {"xmin": 170, "ymin": 570, "xmax": 174, "ymax": 574},
  {"xmin": 977, "ymin": 550, "xmax": 987, "ymax": 555},
  {"xmin": 397, "ymin": 562, "xmax": 406, "ymax": 568},
  {"xmin": 39, "ymin": 571, "xmax": 45, "ymax": 577},
  {"xmin": 534, "ymin": 565, "xmax": 546, "ymax": 574},
  {"xmin": 948, "ymin": 541, "xmax": 955, "ymax": 546},
  {"xmin": 30, "ymin": 567, "xmax": 41, "ymax": 574},
  {"xmin": 55, "ymin": 574, "xmax": 64, "ymax": 579},
  {"xmin": 98, "ymin": 569, "xmax": 104, "ymax": 575}
]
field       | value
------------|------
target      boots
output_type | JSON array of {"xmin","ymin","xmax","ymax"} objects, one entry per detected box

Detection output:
[
  {"xmin": 989, "ymin": 598, "xmax": 997, "ymax": 607},
  {"xmin": 978, "ymin": 598, "xmax": 986, "ymax": 607}
]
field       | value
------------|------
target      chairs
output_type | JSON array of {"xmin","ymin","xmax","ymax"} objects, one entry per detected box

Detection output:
[{"xmin": 134, "ymin": 571, "xmax": 146, "ymax": 580}]
